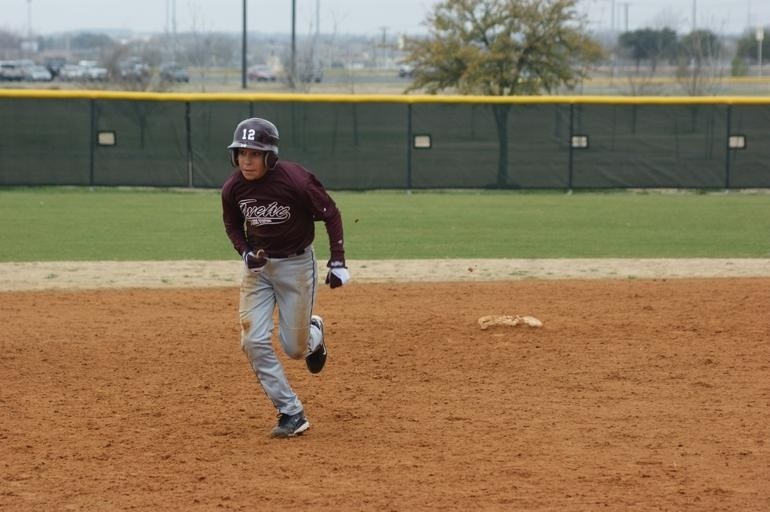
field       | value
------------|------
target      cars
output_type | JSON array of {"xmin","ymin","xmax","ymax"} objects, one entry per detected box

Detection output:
[
  {"xmin": 160, "ymin": 64, "xmax": 190, "ymax": 82},
  {"xmin": 247, "ymin": 65, "xmax": 279, "ymax": 82},
  {"xmin": 283, "ymin": 61, "xmax": 325, "ymax": 83},
  {"xmin": 120, "ymin": 60, "xmax": 150, "ymax": 80},
  {"xmin": 1, "ymin": 58, "xmax": 109, "ymax": 82}
]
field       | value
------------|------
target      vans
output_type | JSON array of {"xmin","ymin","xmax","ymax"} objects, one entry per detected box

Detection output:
[{"xmin": 400, "ymin": 59, "xmax": 437, "ymax": 77}]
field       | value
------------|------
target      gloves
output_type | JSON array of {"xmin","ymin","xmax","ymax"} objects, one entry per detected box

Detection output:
[
  {"xmin": 267, "ymin": 249, "xmax": 305, "ymax": 258},
  {"xmin": 324, "ymin": 264, "xmax": 351, "ymax": 289},
  {"xmin": 241, "ymin": 248, "xmax": 271, "ymax": 276}
]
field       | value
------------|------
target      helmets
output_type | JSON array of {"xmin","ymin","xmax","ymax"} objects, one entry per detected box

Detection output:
[{"xmin": 226, "ymin": 117, "xmax": 280, "ymax": 170}]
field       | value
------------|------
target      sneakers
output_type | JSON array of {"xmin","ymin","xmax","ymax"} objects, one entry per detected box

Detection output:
[
  {"xmin": 269, "ymin": 408, "xmax": 310, "ymax": 439},
  {"xmin": 303, "ymin": 312, "xmax": 328, "ymax": 375}
]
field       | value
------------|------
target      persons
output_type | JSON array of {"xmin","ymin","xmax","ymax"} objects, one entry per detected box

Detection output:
[{"xmin": 221, "ymin": 116, "xmax": 352, "ymax": 440}]
